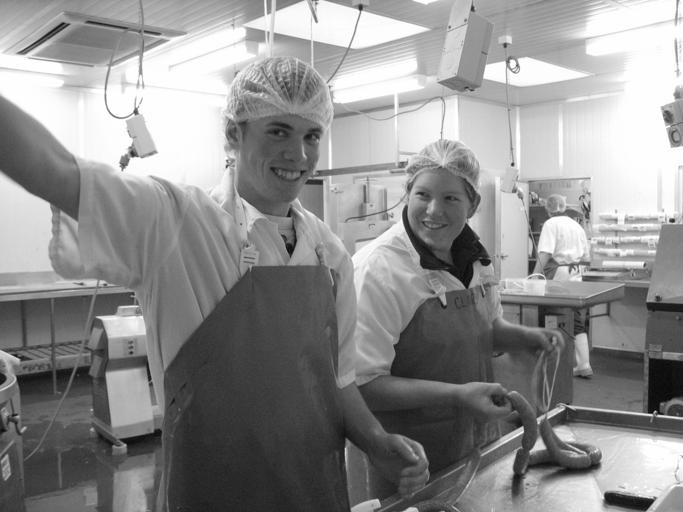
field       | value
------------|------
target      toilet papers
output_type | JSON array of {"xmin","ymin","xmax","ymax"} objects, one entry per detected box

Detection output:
[{"xmin": 600, "ymin": 260, "xmax": 646, "ymax": 270}]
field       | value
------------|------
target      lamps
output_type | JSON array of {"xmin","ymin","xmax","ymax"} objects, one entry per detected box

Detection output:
[{"xmin": 331, "ymin": 73, "xmax": 426, "ymax": 104}]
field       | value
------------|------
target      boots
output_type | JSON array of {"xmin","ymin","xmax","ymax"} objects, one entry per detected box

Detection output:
[{"xmin": 573, "ymin": 332, "xmax": 593, "ymax": 377}]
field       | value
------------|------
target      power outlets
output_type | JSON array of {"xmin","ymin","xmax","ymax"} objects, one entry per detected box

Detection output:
[
  {"xmin": 351, "ymin": 0, "xmax": 370, "ymax": 9},
  {"xmin": 498, "ymin": 36, "xmax": 512, "ymax": 46}
]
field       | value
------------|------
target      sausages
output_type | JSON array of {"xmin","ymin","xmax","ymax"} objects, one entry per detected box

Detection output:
[{"xmin": 402, "ymin": 390, "xmax": 603, "ymax": 512}]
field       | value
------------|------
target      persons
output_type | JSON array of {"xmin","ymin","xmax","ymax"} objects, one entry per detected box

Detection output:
[
  {"xmin": 343, "ymin": 139, "xmax": 569, "ymax": 503},
  {"xmin": 0, "ymin": 54, "xmax": 432, "ymax": 511},
  {"xmin": 528, "ymin": 190, "xmax": 596, "ymax": 381}
]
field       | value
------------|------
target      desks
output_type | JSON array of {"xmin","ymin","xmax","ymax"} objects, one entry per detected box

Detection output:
[
  {"xmin": 500, "ymin": 278, "xmax": 625, "ymax": 336},
  {"xmin": 581, "ymin": 273, "xmax": 651, "ymax": 353},
  {"xmin": 1, "ymin": 279, "xmax": 136, "ymax": 397}
]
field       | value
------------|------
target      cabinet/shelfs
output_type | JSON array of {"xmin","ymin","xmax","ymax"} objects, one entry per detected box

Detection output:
[{"xmin": 529, "ymin": 203, "xmax": 581, "ymax": 276}]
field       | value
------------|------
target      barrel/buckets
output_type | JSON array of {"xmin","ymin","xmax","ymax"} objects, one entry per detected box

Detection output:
[{"xmin": 522, "ymin": 274, "xmax": 547, "ymax": 295}]
